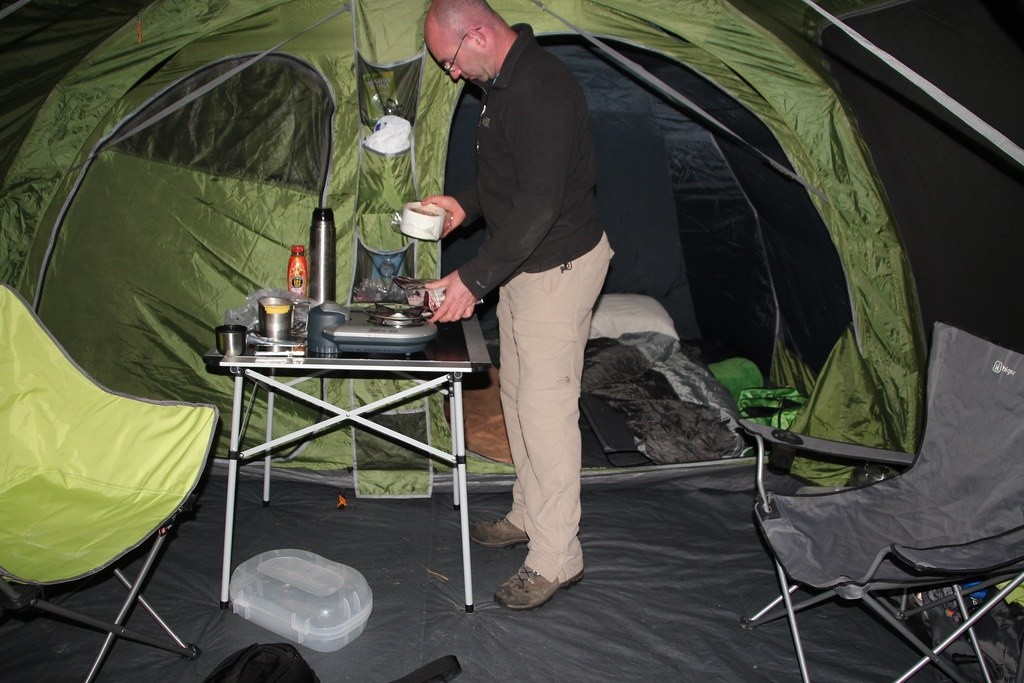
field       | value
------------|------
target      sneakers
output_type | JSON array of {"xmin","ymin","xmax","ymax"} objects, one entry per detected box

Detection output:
[
  {"xmin": 470, "ymin": 518, "xmax": 529, "ymax": 548},
  {"xmin": 493, "ymin": 563, "xmax": 584, "ymax": 609}
]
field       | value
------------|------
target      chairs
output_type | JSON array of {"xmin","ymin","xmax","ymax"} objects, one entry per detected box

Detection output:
[
  {"xmin": 737, "ymin": 318, "xmax": 1024, "ymax": 683},
  {"xmin": 0, "ymin": 279, "xmax": 222, "ymax": 683}
]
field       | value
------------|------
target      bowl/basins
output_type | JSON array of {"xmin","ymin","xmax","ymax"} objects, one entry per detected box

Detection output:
[{"xmin": 405, "ymin": 283, "xmax": 483, "ymax": 314}]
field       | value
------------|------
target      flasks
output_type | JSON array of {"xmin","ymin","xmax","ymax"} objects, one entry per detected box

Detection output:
[{"xmin": 309, "ymin": 206, "xmax": 337, "ymax": 302}]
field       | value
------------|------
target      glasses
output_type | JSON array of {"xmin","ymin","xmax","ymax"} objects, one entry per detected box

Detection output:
[{"xmin": 444, "ymin": 27, "xmax": 481, "ymax": 74}]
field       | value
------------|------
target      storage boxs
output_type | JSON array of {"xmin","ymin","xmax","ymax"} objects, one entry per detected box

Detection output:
[{"xmin": 227, "ymin": 550, "xmax": 380, "ymax": 653}]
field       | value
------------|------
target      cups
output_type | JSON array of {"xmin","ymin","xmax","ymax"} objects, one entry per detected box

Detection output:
[
  {"xmin": 400, "ymin": 201, "xmax": 446, "ymax": 242},
  {"xmin": 847, "ymin": 462, "xmax": 899, "ymax": 488},
  {"xmin": 258, "ymin": 296, "xmax": 293, "ymax": 352},
  {"xmin": 215, "ymin": 325, "xmax": 248, "ymax": 356}
]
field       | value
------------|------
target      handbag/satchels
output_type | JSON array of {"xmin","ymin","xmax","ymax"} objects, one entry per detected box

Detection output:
[
  {"xmin": 736, "ymin": 386, "xmax": 808, "ymax": 456},
  {"xmin": 202, "ymin": 642, "xmax": 462, "ymax": 683}
]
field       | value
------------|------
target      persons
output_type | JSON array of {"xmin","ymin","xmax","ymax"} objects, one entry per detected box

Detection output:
[{"xmin": 421, "ymin": 0, "xmax": 615, "ymax": 608}]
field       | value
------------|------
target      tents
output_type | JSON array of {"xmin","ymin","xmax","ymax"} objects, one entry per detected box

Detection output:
[{"xmin": 0, "ymin": 0, "xmax": 928, "ymax": 496}]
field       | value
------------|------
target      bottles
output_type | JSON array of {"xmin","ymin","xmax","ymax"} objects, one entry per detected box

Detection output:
[{"xmin": 287, "ymin": 244, "xmax": 308, "ymax": 298}]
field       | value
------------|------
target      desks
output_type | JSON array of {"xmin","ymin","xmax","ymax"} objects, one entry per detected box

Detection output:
[{"xmin": 219, "ymin": 290, "xmax": 490, "ymax": 614}]
